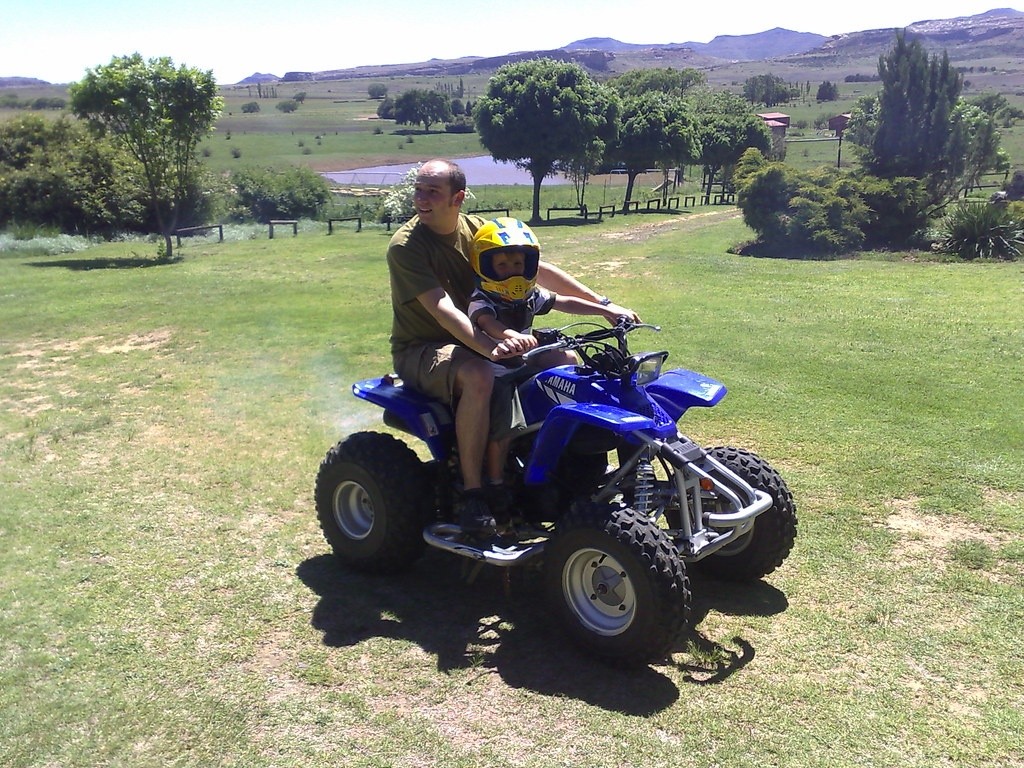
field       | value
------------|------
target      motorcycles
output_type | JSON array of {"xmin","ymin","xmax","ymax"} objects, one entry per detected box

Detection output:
[{"xmin": 313, "ymin": 316, "xmax": 799, "ymax": 672}]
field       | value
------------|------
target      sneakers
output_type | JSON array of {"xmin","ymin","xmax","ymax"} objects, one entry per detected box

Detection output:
[{"xmin": 461, "ymin": 492, "xmax": 496, "ymax": 532}]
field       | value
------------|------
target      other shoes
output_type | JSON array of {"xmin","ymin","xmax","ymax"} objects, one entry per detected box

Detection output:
[{"xmin": 493, "ymin": 504, "xmax": 511, "ymax": 535}]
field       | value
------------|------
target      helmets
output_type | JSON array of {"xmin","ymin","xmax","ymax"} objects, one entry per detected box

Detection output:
[{"xmin": 470, "ymin": 216, "xmax": 541, "ymax": 300}]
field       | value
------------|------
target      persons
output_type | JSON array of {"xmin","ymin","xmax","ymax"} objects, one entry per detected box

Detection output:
[
  {"xmin": 468, "ymin": 217, "xmax": 635, "ymax": 525},
  {"xmin": 386, "ymin": 158, "xmax": 644, "ymax": 543}
]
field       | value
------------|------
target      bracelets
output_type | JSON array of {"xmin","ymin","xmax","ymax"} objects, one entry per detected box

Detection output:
[{"xmin": 599, "ymin": 295, "xmax": 613, "ymax": 306}]
